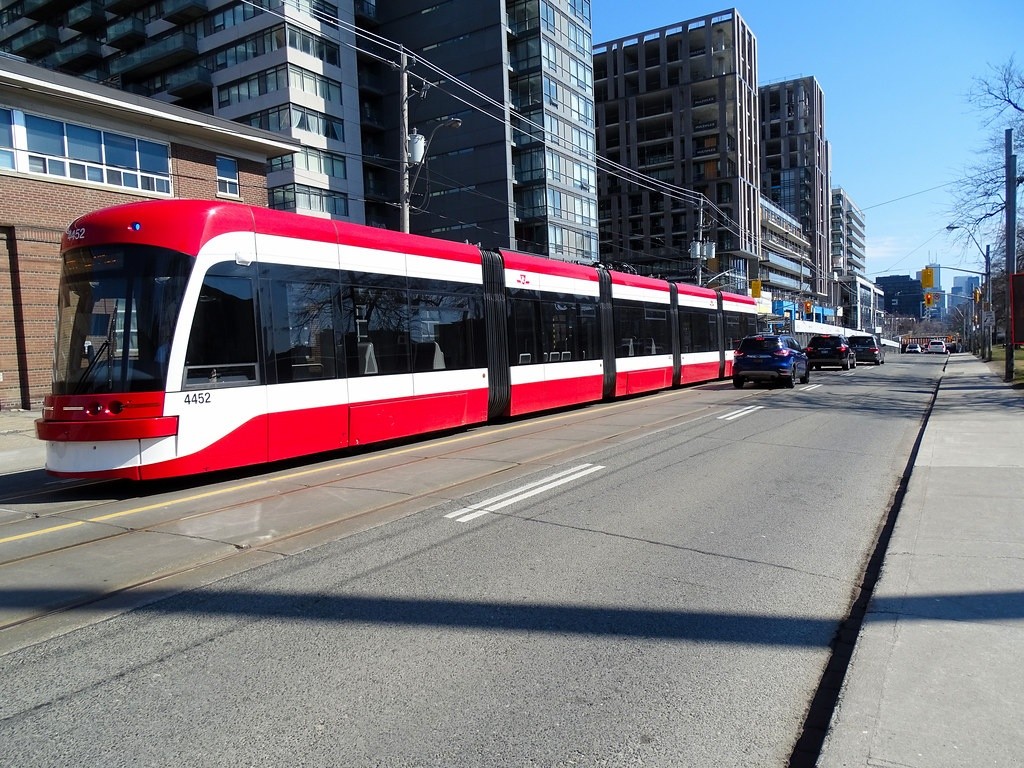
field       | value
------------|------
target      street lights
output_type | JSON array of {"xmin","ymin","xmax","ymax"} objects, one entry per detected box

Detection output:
[
  {"xmin": 946, "ymin": 226, "xmax": 993, "ymax": 360},
  {"xmin": 397, "ymin": 117, "xmax": 463, "ymax": 235}
]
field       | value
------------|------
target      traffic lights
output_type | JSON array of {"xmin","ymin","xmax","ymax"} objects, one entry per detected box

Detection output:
[
  {"xmin": 925, "ymin": 294, "xmax": 934, "ymax": 306},
  {"xmin": 805, "ymin": 301, "xmax": 812, "ymax": 314},
  {"xmin": 974, "ymin": 289, "xmax": 980, "ymax": 303}
]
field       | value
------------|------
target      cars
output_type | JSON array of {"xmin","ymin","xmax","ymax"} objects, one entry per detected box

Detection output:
[
  {"xmin": 849, "ymin": 334, "xmax": 892, "ymax": 363},
  {"xmin": 806, "ymin": 335, "xmax": 858, "ymax": 371},
  {"xmin": 905, "ymin": 339, "xmax": 956, "ymax": 354},
  {"xmin": 732, "ymin": 334, "xmax": 812, "ymax": 389}
]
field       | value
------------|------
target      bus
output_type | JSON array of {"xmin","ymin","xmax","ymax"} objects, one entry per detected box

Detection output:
[{"xmin": 32, "ymin": 198, "xmax": 760, "ymax": 482}]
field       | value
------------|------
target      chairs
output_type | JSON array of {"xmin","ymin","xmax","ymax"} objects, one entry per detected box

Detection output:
[{"xmin": 348, "ymin": 336, "xmax": 736, "ymax": 377}]
